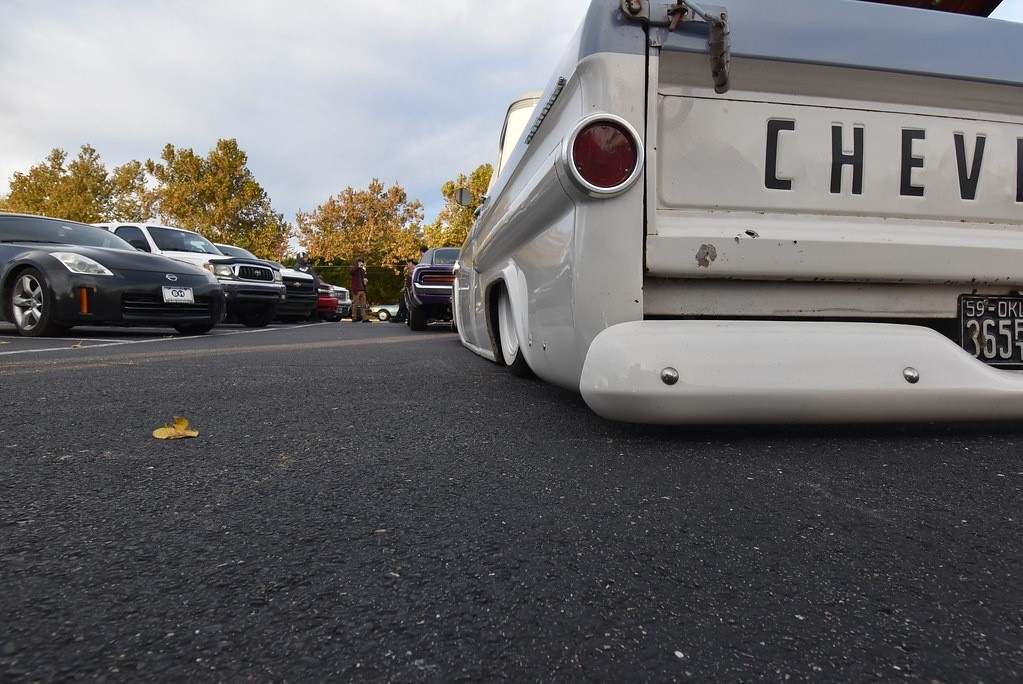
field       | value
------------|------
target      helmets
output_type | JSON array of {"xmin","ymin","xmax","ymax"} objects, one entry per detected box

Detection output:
[{"xmin": 355, "ymin": 257, "xmax": 364, "ymax": 266}]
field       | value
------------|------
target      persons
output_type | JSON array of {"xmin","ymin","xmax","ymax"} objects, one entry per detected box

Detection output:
[
  {"xmin": 294, "ymin": 252, "xmax": 326, "ymax": 322},
  {"xmin": 387, "ymin": 245, "xmax": 429, "ymax": 324},
  {"xmin": 347, "ymin": 256, "xmax": 373, "ymax": 323}
]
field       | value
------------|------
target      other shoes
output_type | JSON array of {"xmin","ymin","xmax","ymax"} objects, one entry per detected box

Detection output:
[
  {"xmin": 352, "ymin": 319, "xmax": 356, "ymax": 323},
  {"xmin": 362, "ymin": 319, "xmax": 372, "ymax": 323}
]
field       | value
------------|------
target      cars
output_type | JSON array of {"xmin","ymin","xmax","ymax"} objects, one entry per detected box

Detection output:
[
  {"xmin": 369, "ymin": 304, "xmax": 401, "ymax": 323},
  {"xmin": 66, "ymin": 221, "xmax": 353, "ymax": 330},
  {"xmin": 451, "ymin": 1, "xmax": 1022, "ymax": 424},
  {"xmin": 0, "ymin": 212, "xmax": 227, "ymax": 339},
  {"xmin": 407, "ymin": 246, "xmax": 463, "ymax": 331}
]
